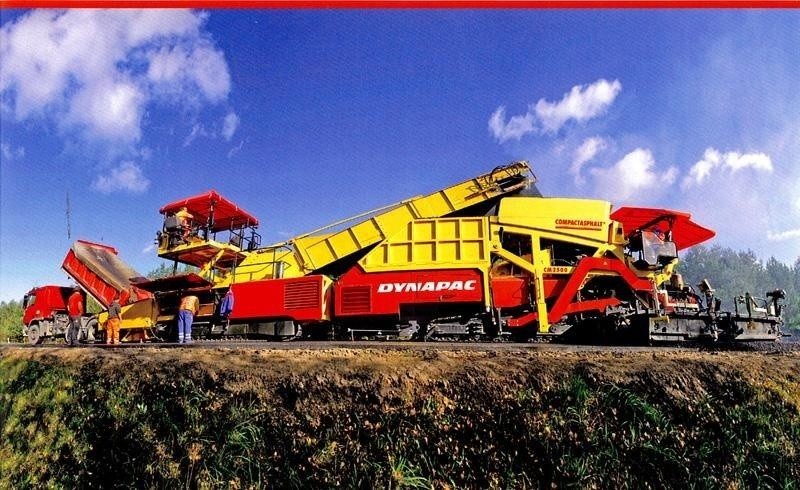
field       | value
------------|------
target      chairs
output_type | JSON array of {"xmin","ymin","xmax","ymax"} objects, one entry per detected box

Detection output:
[{"xmin": 164, "ymin": 218, "xmax": 183, "ymax": 249}]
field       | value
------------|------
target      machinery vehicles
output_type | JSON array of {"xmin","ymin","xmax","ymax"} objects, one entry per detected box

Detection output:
[{"xmin": 98, "ymin": 159, "xmax": 793, "ymax": 345}]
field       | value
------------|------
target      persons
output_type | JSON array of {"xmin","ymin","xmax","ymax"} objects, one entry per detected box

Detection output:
[
  {"xmin": 178, "ymin": 295, "xmax": 199, "ymax": 343},
  {"xmin": 67, "ymin": 286, "xmax": 83, "ymax": 345},
  {"xmin": 106, "ymin": 296, "xmax": 123, "ymax": 345},
  {"xmin": 173, "ymin": 207, "xmax": 194, "ymax": 242},
  {"xmin": 652, "ymin": 227, "xmax": 665, "ymax": 254}
]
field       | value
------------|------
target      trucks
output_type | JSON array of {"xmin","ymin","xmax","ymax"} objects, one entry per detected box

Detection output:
[{"xmin": 20, "ymin": 239, "xmax": 152, "ymax": 346}]
